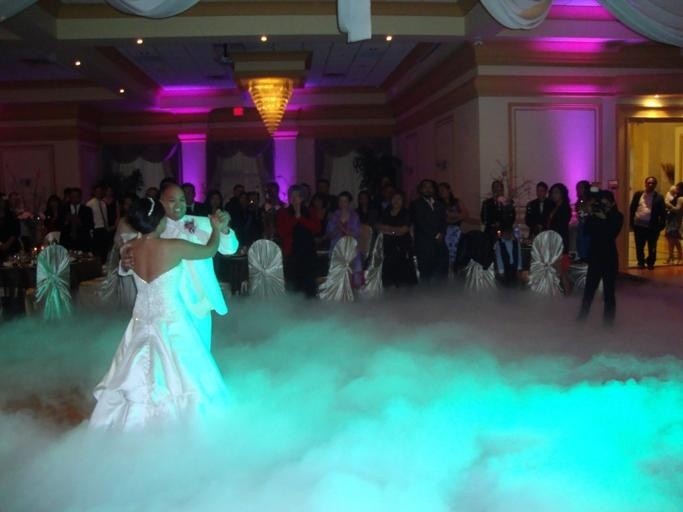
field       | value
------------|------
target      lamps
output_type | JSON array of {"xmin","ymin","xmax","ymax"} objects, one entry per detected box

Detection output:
[{"xmin": 231, "ymin": 54, "xmax": 314, "ymax": 141}]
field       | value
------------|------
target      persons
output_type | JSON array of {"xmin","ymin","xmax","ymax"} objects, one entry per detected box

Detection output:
[
  {"xmin": 92, "ymin": 197, "xmax": 226, "ymax": 458},
  {"xmin": 2, "ymin": 186, "xmax": 165, "ymax": 296},
  {"xmin": 115, "ymin": 183, "xmax": 239, "ymax": 360},
  {"xmin": 628, "ymin": 177, "xmax": 682, "ymax": 271},
  {"xmin": 480, "ymin": 180, "xmax": 625, "ymax": 322},
  {"xmin": 182, "ymin": 179, "xmax": 468, "ymax": 300}
]
